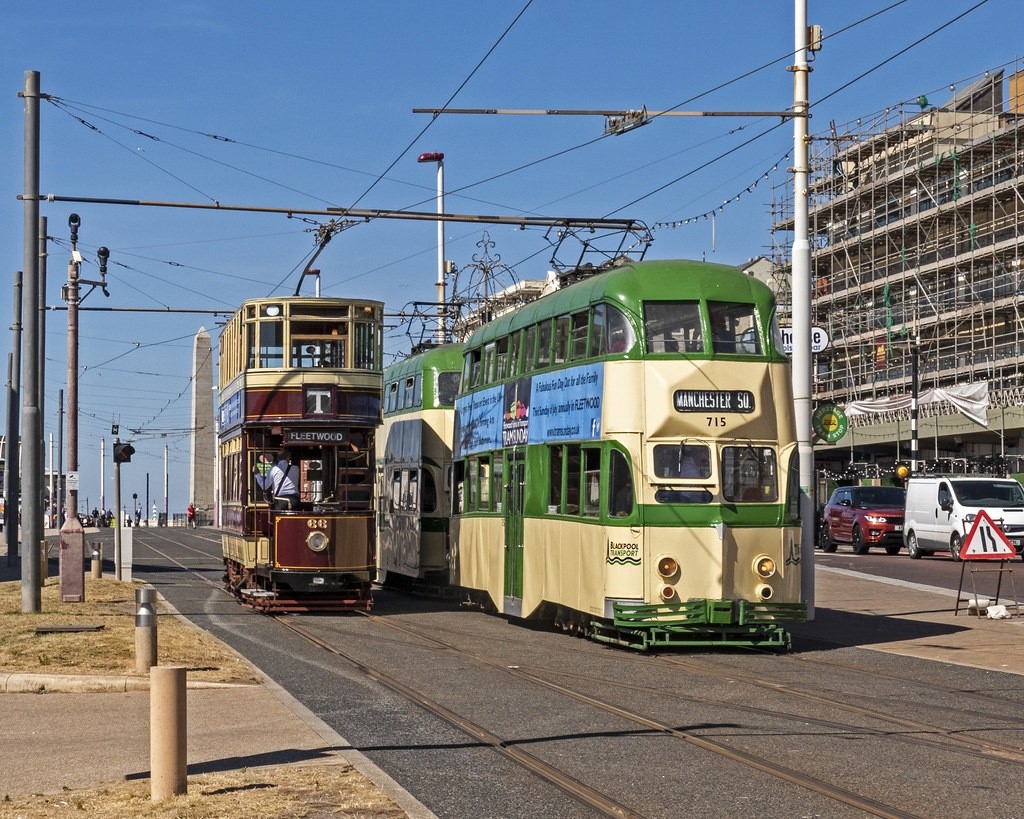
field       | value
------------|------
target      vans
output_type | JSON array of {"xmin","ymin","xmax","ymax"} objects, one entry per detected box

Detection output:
[{"xmin": 903, "ymin": 477, "xmax": 1024, "ymax": 562}]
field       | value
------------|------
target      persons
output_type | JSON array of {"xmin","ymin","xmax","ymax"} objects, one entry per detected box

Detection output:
[
  {"xmin": 136, "ymin": 507, "xmax": 142, "ymax": 527},
  {"xmin": 187, "ymin": 503, "xmax": 196, "ymax": 530},
  {"xmin": 611, "ymin": 328, "xmax": 626, "ymax": 352},
  {"xmin": 251, "ymin": 449, "xmax": 301, "ymax": 509},
  {"xmin": 127, "ymin": 517, "xmax": 132, "ymax": 527},
  {"xmin": 92, "ymin": 507, "xmax": 99, "ymax": 524},
  {"xmin": 100, "ymin": 508, "xmax": 106, "ymax": 526},
  {"xmin": 681, "ymin": 447, "xmax": 707, "ymax": 502},
  {"xmin": 107, "ymin": 509, "xmax": 113, "ymax": 526}
]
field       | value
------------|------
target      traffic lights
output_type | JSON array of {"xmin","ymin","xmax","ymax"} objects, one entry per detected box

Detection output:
[
  {"xmin": 133, "ymin": 494, "xmax": 137, "ymax": 498},
  {"xmin": 113, "ymin": 444, "xmax": 136, "ymax": 464}
]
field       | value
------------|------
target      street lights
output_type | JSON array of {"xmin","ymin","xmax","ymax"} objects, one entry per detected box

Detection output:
[
  {"xmin": 60, "ymin": 212, "xmax": 111, "ymax": 602},
  {"xmin": 417, "ymin": 152, "xmax": 448, "ymax": 346}
]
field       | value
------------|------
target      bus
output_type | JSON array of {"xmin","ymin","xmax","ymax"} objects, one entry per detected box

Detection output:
[
  {"xmin": 215, "ymin": 295, "xmax": 385, "ymax": 613},
  {"xmin": 376, "ymin": 260, "xmax": 804, "ymax": 654}
]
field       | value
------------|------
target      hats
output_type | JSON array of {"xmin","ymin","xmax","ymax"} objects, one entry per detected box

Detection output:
[{"xmin": 190, "ymin": 503, "xmax": 193, "ymax": 506}]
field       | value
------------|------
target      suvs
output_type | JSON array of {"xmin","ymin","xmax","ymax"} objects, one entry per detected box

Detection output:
[{"xmin": 821, "ymin": 486, "xmax": 906, "ymax": 555}]
